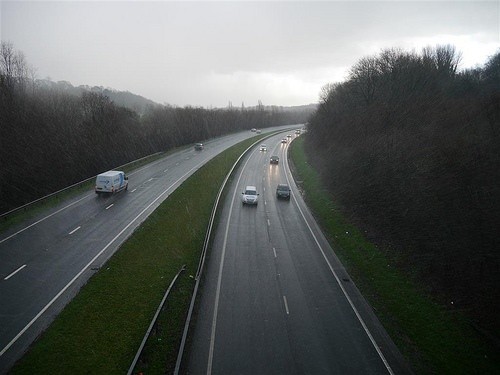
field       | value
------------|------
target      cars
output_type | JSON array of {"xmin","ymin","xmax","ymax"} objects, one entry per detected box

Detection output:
[
  {"xmin": 287, "ymin": 133, "xmax": 293, "ymax": 137},
  {"xmin": 259, "ymin": 143, "xmax": 267, "ymax": 151},
  {"xmin": 256, "ymin": 129, "xmax": 262, "ymax": 134},
  {"xmin": 270, "ymin": 156, "xmax": 280, "ymax": 165},
  {"xmin": 250, "ymin": 127, "xmax": 257, "ymax": 132},
  {"xmin": 282, "ymin": 138, "xmax": 287, "ymax": 143},
  {"xmin": 277, "ymin": 184, "xmax": 292, "ymax": 201}
]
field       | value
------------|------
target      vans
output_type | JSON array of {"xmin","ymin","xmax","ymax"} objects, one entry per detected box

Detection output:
[
  {"xmin": 295, "ymin": 129, "xmax": 301, "ymax": 134},
  {"xmin": 195, "ymin": 142, "xmax": 203, "ymax": 151},
  {"xmin": 242, "ymin": 185, "xmax": 259, "ymax": 208}
]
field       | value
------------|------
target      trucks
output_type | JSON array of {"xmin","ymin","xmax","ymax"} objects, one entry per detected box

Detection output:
[{"xmin": 95, "ymin": 170, "xmax": 129, "ymax": 195}]
